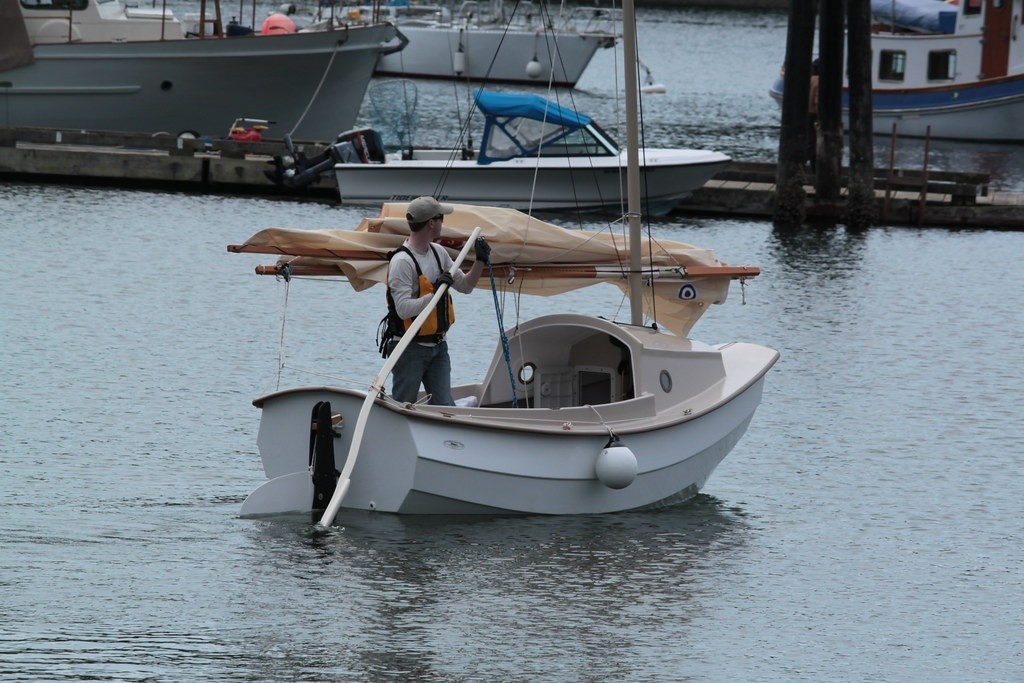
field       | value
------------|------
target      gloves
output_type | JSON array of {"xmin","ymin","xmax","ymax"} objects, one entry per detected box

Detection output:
[
  {"xmin": 475, "ymin": 237, "xmax": 491, "ymax": 265},
  {"xmin": 433, "ymin": 272, "xmax": 454, "ymax": 298}
]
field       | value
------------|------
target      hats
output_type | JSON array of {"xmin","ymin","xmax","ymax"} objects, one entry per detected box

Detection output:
[{"xmin": 408, "ymin": 197, "xmax": 454, "ymax": 224}]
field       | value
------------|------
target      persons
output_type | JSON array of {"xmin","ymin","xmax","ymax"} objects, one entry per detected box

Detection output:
[
  {"xmin": 808, "ymin": 58, "xmax": 820, "ymax": 190},
  {"xmin": 377, "ymin": 195, "xmax": 492, "ymax": 407}
]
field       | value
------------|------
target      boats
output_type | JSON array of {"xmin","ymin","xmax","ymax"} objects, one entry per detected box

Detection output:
[
  {"xmin": 274, "ymin": 80, "xmax": 738, "ymax": 223},
  {"xmin": 766, "ymin": 0, "xmax": 1024, "ymax": 146},
  {"xmin": 1, "ymin": 0, "xmax": 412, "ymax": 151},
  {"xmin": 300, "ymin": 0, "xmax": 615, "ymax": 90}
]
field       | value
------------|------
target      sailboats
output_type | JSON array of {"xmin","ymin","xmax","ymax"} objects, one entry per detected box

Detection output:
[{"xmin": 233, "ymin": 0, "xmax": 787, "ymax": 525}]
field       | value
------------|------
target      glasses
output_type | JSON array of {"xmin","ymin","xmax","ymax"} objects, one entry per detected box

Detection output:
[{"xmin": 432, "ymin": 214, "xmax": 444, "ymax": 222}]
